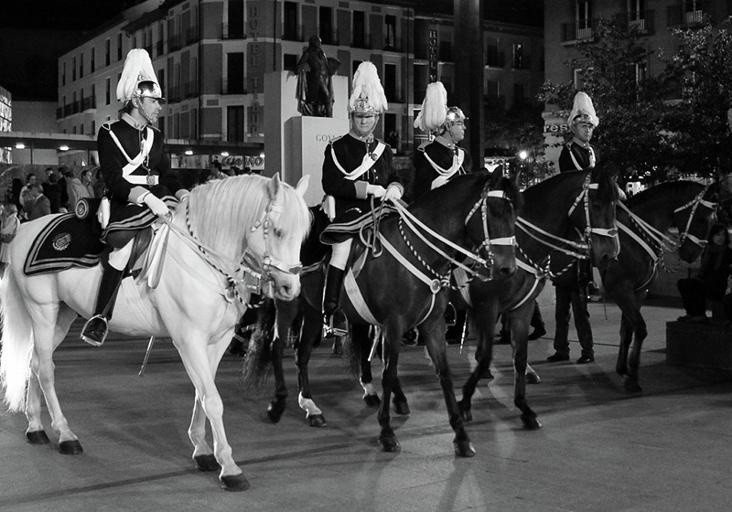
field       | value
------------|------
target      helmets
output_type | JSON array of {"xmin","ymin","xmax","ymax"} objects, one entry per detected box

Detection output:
[
  {"xmin": 347, "ymin": 95, "xmax": 382, "ymax": 118},
  {"xmin": 571, "ymin": 111, "xmax": 595, "ymax": 127},
  {"xmin": 129, "ymin": 80, "xmax": 167, "ymax": 104},
  {"xmin": 428, "ymin": 106, "xmax": 467, "ymax": 136}
]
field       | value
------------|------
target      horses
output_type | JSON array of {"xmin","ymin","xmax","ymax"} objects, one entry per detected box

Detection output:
[
  {"xmin": 474, "ymin": 176, "xmax": 732, "ymax": 392},
  {"xmin": 331, "ymin": 156, "xmax": 622, "ymax": 430},
  {"xmin": 238, "ymin": 164, "xmax": 529, "ymax": 458},
  {"xmin": 0, "ymin": 171, "xmax": 312, "ymax": 491}
]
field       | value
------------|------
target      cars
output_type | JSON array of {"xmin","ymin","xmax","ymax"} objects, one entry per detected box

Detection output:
[{"xmin": 586, "ymin": 174, "xmax": 732, "ymax": 307}]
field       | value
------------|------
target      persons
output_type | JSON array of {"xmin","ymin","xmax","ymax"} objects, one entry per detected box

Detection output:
[
  {"xmin": 204, "ymin": 159, "xmax": 229, "ymax": 181},
  {"xmin": 676, "ymin": 224, "xmax": 731, "ymax": 322},
  {"xmin": 496, "ymin": 302, "xmax": 546, "ymax": 344},
  {"xmin": 412, "ymin": 119, "xmax": 475, "ymax": 202},
  {"xmin": 82, "ymin": 95, "xmax": 192, "ymax": 344},
  {"xmin": 296, "ymin": 35, "xmax": 336, "ymax": 118},
  {"xmin": 164, "ymin": 172, "xmax": 181, "ymax": 195},
  {"xmin": 228, "ymin": 166, "xmax": 239, "ymax": 177},
  {"xmin": 320, "ymin": 110, "xmax": 407, "ymax": 330},
  {"xmin": 545, "ymin": 277, "xmax": 598, "ymax": 365},
  {"xmin": 558, "ymin": 120, "xmax": 631, "ymax": 206},
  {"xmin": 0, "ymin": 163, "xmax": 107, "ymax": 270}
]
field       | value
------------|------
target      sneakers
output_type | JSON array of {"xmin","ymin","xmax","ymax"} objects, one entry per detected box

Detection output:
[{"xmin": 529, "ymin": 328, "xmax": 547, "ymax": 341}]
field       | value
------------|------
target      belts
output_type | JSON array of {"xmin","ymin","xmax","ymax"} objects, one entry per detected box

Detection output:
[{"xmin": 122, "ymin": 174, "xmax": 161, "ymax": 187}]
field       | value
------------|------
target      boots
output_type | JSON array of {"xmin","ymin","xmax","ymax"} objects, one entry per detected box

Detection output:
[
  {"xmin": 325, "ymin": 263, "xmax": 347, "ymax": 329},
  {"xmin": 81, "ymin": 261, "xmax": 124, "ymax": 343}
]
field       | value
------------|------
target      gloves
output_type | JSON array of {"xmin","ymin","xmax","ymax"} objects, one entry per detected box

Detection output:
[
  {"xmin": 382, "ymin": 184, "xmax": 402, "ymax": 201},
  {"xmin": 143, "ymin": 192, "xmax": 170, "ymax": 216},
  {"xmin": 431, "ymin": 175, "xmax": 450, "ymax": 191},
  {"xmin": 367, "ymin": 183, "xmax": 387, "ymax": 198},
  {"xmin": 175, "ymin": 189, "xmax": 190, "ymax": 201}
]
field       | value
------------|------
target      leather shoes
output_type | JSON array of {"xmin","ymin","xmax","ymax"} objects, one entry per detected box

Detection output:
[
  {"xmin": 576, "ymin": 354, "xmax": 595, "ymax": 364},
  {"xmin": 545, "ymin": 352, "xmax": 571, "ymax": 363}
]
